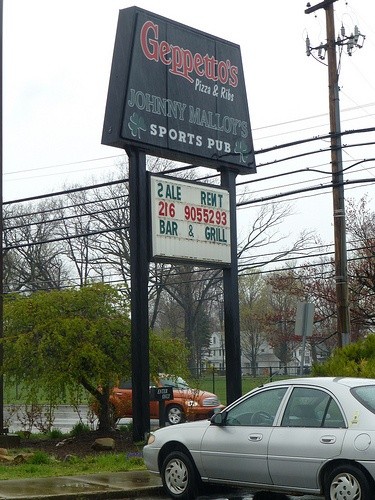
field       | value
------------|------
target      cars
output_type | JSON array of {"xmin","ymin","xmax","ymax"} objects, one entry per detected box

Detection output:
[
  {"xmin": 87, "ymin": 373, "xmax": 226, "ymax": 425},
  {"xmin": 144, "ymin": 377, "xmax": 375, "ymax": 500}
]
